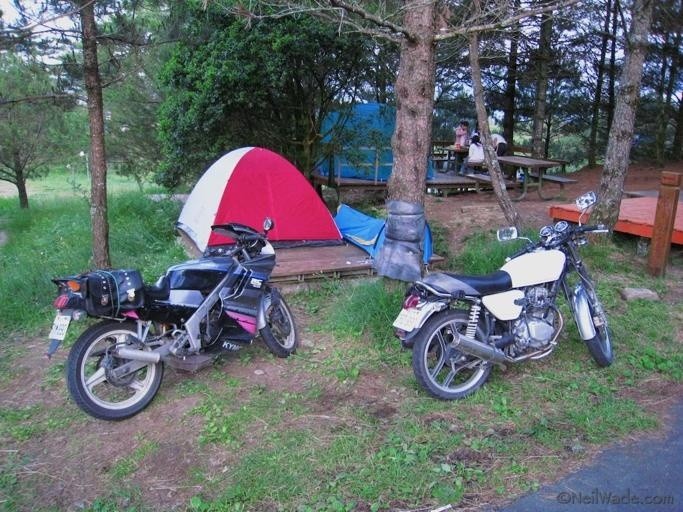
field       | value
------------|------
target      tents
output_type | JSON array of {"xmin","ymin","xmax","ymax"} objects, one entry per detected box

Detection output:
[
  {"xmin": 315, "ymin": 101, "xmax": 436, "ymax": 181},
  {"xmin": 176, "ymin": 147, "xmax": 346, "ymax": 256}
]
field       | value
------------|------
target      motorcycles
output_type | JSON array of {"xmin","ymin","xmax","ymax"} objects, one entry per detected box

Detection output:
[
  {"xmin": 45, "ymin": 216, "xmax": 297, "ymax": 422},
  {"xmin": 393, "ymin": 191, "xmax": 613, "ymax": 401}
]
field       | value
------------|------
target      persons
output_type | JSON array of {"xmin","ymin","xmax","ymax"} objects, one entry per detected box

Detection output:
[
  {"xmin": 459, "ymin": 136, "xmax": 484, "ymax": 176},
  {"xmin": 454, "ymin": 121, "xmax": 469, "ymax": 146},
  {"xmin": 490, "ymin": 133, "xmax": 508, "ymax": 167}
]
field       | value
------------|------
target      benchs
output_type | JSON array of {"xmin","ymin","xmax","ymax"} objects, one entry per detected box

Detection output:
[{"xmin": 466, "ymin": 172, "xmax": 578, "ymax": 203}]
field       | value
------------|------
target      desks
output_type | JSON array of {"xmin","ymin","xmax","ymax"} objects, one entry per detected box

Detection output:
[
  {"xmin": 496, "ymin": 155, "xmax": 561, "ymax": 201},
  {"xmin": 442, "ymin": 146, "xmax": 469, "ymax": 176}
]
